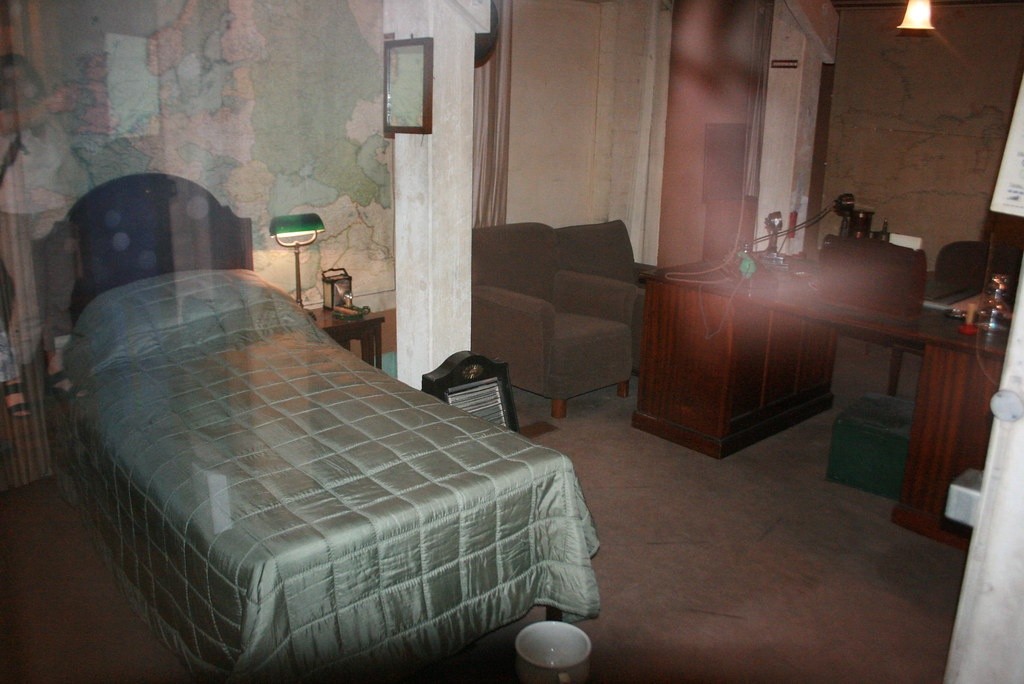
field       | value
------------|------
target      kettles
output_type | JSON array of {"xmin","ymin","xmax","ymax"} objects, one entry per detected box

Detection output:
[{"xmin": 849, "ymin": 209, "xmax": 875, "ymax": 240}]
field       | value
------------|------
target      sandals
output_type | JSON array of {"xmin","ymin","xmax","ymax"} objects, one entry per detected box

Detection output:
[
  {"xmin": 46, "ymin": 371, "xmax": 77, "ymax": 403},
  {"xmin": 5, "ymin": 379, "xmax": 30, "ymax": 420}
]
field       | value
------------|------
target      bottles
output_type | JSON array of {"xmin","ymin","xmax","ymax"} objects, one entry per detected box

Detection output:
[
  {"xmin": 877, "ymin": 219, "xmax": 889, "ymax": 241},
  {"xmin": 0, "ymin": 317, "xmax": 19, "ymax": 383},
  {"xmin": 977, "ymin": 273, "xmax": 1011, "ymax": 336}
]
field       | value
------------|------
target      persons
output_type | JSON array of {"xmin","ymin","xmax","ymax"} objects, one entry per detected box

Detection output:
[{"xmin": 0, "ymin": 52, "xmax": 80, "ymax": 419}]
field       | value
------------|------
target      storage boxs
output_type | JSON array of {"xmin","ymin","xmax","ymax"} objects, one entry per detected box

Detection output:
[{"xmin": 824, "ymin": 395, "xmax": 915, "ymax": 501}]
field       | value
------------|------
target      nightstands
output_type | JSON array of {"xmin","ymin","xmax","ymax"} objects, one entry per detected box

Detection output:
[{"xmin": 310, "ymin": 307, "xmax": 385, "ymax": 370}]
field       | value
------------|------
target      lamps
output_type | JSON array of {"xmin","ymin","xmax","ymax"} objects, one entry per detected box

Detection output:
[
  {"xmin": 896, "ymin": 0, "xmax": 935, "ymax": 29},
  {"xmin": 269, "ymin": 213, "xmax": 326, "ymax": 321}
]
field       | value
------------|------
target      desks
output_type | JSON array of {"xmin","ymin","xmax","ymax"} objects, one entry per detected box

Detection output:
[{"xmin": 630, "ymin": 252, "xmax": 1018, "ymax": 551}]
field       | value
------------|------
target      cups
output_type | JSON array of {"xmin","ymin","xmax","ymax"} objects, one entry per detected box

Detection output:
[{"xmin": 515, "ymin": 620, "xmax": 591, "ymax": 684}]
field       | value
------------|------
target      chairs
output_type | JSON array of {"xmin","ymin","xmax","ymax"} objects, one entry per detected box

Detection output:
[{"xmin": 886, "ymin": 240, "xmax": 1022, "ymax": 397}]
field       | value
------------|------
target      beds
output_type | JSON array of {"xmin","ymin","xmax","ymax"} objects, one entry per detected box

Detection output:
[{"xmin": 29, "ymin": 173, "xmax": 600, "ymax": 684}]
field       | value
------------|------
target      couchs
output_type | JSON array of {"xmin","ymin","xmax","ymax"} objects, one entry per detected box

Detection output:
[
  {"xmin": 472, "ymin": 222, "xmax": 639, "ymax": 419},
  {"xmin": 553, "ymin": 219, "xmax": 646, "ymax": 377}
]
